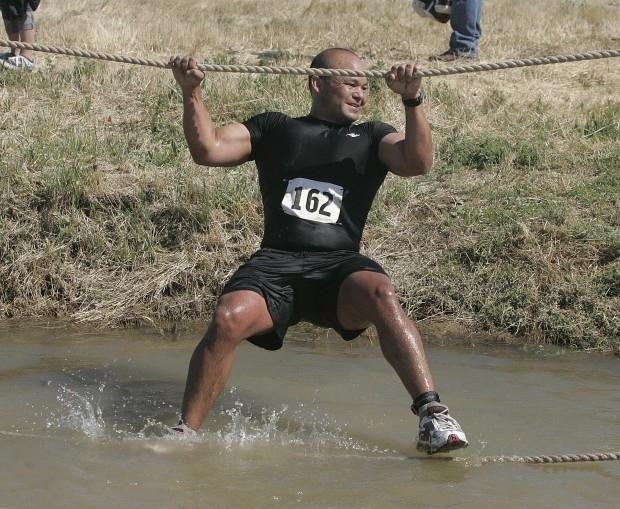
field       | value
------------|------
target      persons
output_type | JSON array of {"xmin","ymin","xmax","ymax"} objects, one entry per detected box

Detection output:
[
  {"xmin": 168, "ymin": 45, "xmax": 472, "ymax": 457},
  {"xmin": 408, "ymin": 0, "xmax": 485, "ymax": 61},
  {"xmin": 1, "ymin": 0, "xmax": 43, "ymax": 72}
]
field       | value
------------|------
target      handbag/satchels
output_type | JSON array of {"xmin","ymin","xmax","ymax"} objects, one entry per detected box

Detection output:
[{"xmin": 412, "ymin": 0, "xmax": 452, "ymax": 23}]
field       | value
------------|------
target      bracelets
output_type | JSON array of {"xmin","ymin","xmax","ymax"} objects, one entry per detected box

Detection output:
[{"xmin": 401, "ymin": 91, "xmax": 425, "ymax": 108}]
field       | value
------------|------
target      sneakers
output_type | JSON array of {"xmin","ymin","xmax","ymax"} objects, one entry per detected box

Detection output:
[
  {"xmin": 428, "ymin": 50, "xmax": 478, "ymax": 63},
  {"xmin": 415, "ymin": 401, "xmax": 469, "ymax": 453},
  {"xmin": 0, "ymin": 55, "xmax": 35, "ymax": 73}
]
